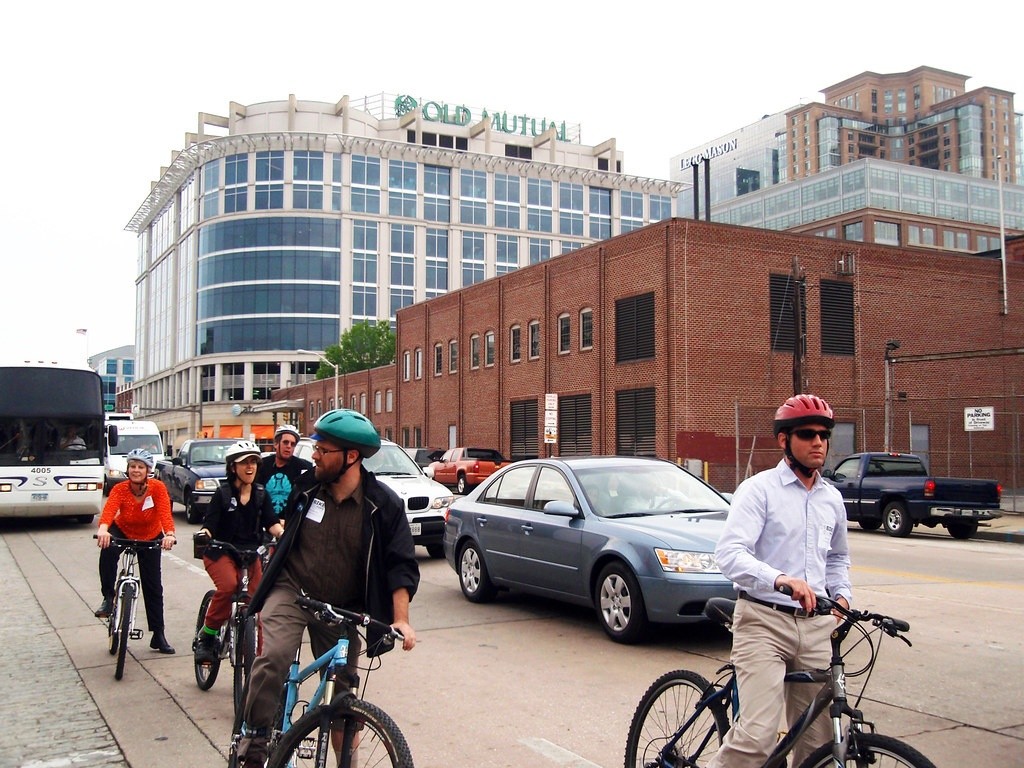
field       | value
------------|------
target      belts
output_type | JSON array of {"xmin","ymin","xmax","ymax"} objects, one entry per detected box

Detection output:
[{"xmin": 739, "ymin": 591, "xmax": 821, "ymax": 618}]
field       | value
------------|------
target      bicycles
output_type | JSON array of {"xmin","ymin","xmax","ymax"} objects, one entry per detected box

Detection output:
[
  {"xmin": 228, "ymin": 591, "xmax": 421, "ymax": 768},
  {"xmin": 92, "ymin": 533, "xmax": 177, "ymax": 681},
  {"xmin": 623, "ymin": 582, "xmax": 937, "ymax": 768},
  {"xmin": 190, "ymin": 531, "xmax": 282, "ymax": 722}
]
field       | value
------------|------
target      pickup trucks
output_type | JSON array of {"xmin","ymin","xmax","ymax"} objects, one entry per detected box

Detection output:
[
  {"xmin": 153, "ymin": 438, "xmax": 249, "ymax": 524},
  {"xmin": 822, "ymin": 451, "xmax": 1004, "ymax": 540},
  {"xmin": 426, "ymin": 447, "xmax": 519, "ymax": 496}
]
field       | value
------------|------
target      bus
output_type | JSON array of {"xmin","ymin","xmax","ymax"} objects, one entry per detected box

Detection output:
[{"xmin": 0, "ymin": 359, "xmax": 118, "ymax": 525}]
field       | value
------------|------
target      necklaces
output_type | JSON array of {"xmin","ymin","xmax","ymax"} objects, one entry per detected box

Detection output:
[{"xmin": 129, "ymin": 481, "xmax": 148, "ymax": 497}]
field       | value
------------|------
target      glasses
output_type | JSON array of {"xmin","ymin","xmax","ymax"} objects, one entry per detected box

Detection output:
[
  {"xmin": 311, "ymin": 445, "xmax": 343, "ymax": 456},
  {"xmin": 785, "ymin": 428, "xmax": 832, "ymax": 441},
  {"xmin": 278, "ymin": 440, "xmax": 297, "ymax": 448}
]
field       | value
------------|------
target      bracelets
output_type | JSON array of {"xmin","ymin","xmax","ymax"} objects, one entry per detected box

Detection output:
[
  {"xmin": 277, "ymin": 530, "xmax": 285, "ymax": 537},
  {"xmin": 166, "ymin": 533, "xmax": 176, "ymax": 538}
]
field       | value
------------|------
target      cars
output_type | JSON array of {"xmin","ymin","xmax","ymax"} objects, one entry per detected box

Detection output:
[
  {"xmin": 444, "ymin": 454, "xmax": 743, "ymax": 644},
  {"xmin": 402, "ymin": 447, "xmax": 448, "ymax": 474}
]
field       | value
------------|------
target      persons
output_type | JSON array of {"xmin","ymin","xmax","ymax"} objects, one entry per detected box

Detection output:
[
  {"xmin": 139, "ymin": 438, "xmax": 158, "ymax": 454},
  {"xmin": 95, "ymin": 448, "xmax": 177, "ymax": 654},
  {"xmin": 237, "ymin": 409, "xmax": 420, "ymax": 768},
  {"xmin": 60, "ymin": 425, "xmax": 86, "ymax": 451},
  {"xmin": 259, "ymin": 426, "xmax": 313, "ymax": 517},
  {"xmin": 195, "ymin": 441, "xmax": 286, "ymax": 664},
  {"xmin": 624, "ymin": 473, "xmax": 688, "ymax": 513},
  {"xmin": 706, "ymin": 395, "xmax": 853, "ymax": 768}
]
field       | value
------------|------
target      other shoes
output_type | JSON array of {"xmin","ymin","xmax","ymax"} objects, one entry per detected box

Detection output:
[
  {"xmin": 93, "ymin": 598, "xmax": 113, "ymax": 617},
  {"xmin": 151, "ymin": 634, "xmax": 176, "ymax": 654},
  {"xmin": 238, "ymin": 736, "xmax": 266, "ymax": 768}
]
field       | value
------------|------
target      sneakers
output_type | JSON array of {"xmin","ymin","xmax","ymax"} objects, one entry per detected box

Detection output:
[{"xmin": 196, "ymin": 630, "xmax": 216, "ymax": 662}]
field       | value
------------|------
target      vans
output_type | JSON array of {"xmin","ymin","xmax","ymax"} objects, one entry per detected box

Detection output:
[{"xmin": 103, "ymin": 412, "xmax": 173, "ymax": 496}]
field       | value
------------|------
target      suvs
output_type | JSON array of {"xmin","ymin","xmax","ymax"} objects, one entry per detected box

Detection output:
[{"xmin": 290, "ymin": 435, "xmax": 454, "ymax": 560}]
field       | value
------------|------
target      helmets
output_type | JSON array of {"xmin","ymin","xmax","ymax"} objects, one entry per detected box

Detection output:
[
  {"xmin": 312, "ymin": 409, "xmax": 380, "ymax": 458},
  {"xmin": 225, "ymin": 440, "xmax": 263, "ymax": 461},
  {"xmin": 127, "ymin": 448, "xmax": 155, "ymax": 468},
  {"xmin": 275, "ymin": 424, "xmax": 301, "ymax": 442},
  {"xmin": 774, "ymin": 394, "xmax": 835, "ymax": 425}
]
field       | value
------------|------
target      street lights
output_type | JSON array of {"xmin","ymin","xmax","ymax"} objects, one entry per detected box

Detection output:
[{"xmin": 297, "ymin": 348, "xmax": 338, "ymax": 409}]
